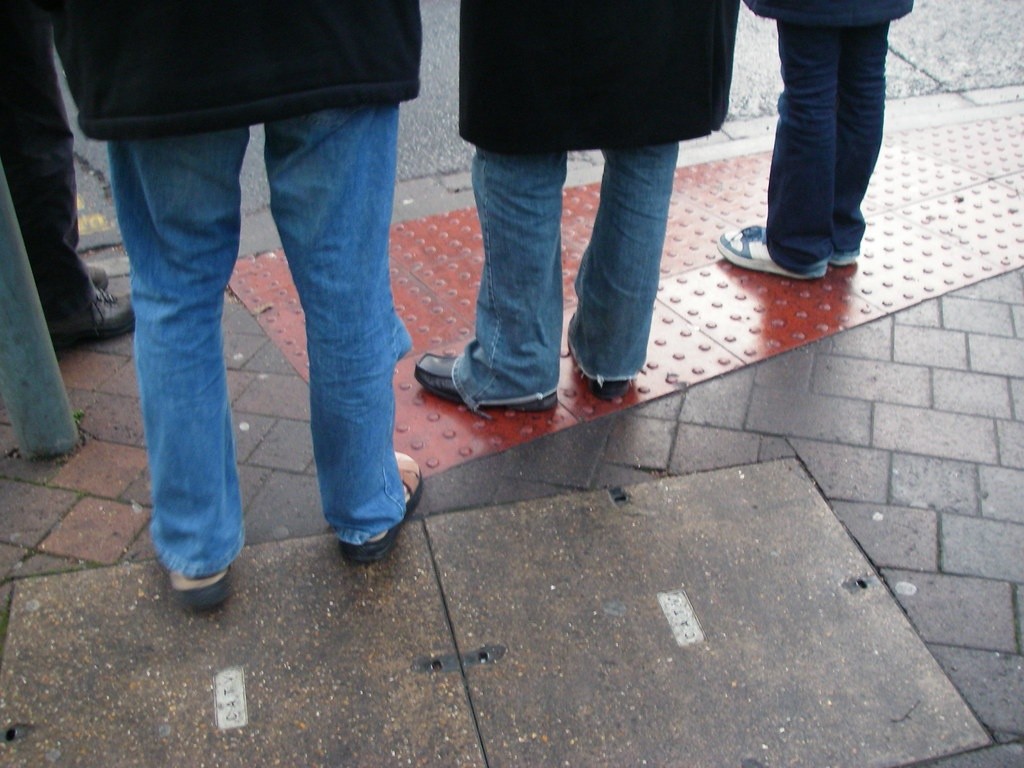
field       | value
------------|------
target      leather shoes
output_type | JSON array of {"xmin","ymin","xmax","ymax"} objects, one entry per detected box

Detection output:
[
  {"xmin": 568, "ymin": 313, "xmax": 630, "ymax": 400},
  {"xmin": 414, "ymin": 352, "xmax": 557, "ymax": 409}
]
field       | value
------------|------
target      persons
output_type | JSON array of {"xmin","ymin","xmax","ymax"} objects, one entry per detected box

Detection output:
[
  {"xmin": 414, "ymin": 0, "xmax": 742, "ymax": 421},
  {"xmin": 46, "ymin": 0, "xmax": 424, "ymax": 607},
  {"xmin": 0, "ymin": 0, "xmax": 136, "ymax": 347},
  {"xmin": 716, "ymin": 0, "xmax": 915, "ymax": 279}
]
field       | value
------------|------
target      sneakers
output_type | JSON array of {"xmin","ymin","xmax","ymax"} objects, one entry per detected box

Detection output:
[
  {"xmin": 717, "ymin": 224, "xmax": 833, "ymax": 279},
  {"xmin": 47, "ymin": 288, "xmax": 135, "ymax": 348},
  {"xmin": 829, "ymin": 249, "xmax": 860, "ymax": 266},
  {"xmin": 89, "ymin": 266, "xmax": 109, "ymax": 291}
]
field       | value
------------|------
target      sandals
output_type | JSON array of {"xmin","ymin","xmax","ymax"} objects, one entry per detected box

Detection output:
[
  {"xmin": 169, "ymin": 562, "xmax": 233, "ymax": 610},
  {"xmin": 339, "ymin": 450, "xmax": 422, "ymax": 561}
]
